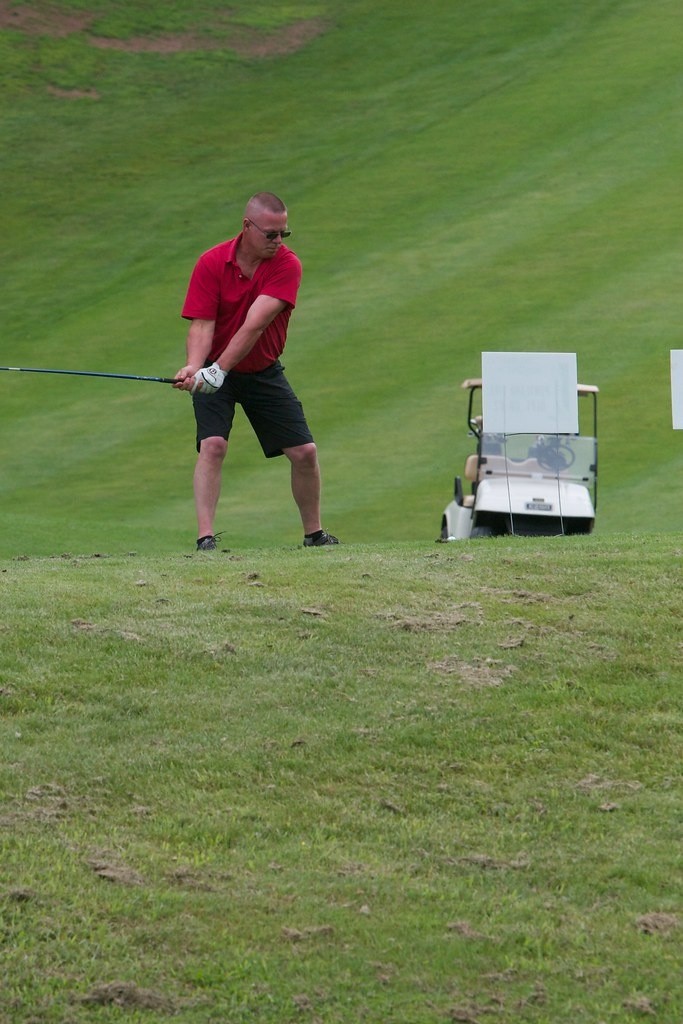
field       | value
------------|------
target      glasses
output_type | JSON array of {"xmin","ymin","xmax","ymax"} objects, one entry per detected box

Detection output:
[{"xmin": 248, "ymin": 218, "xmax": 292, "ymax": 240}]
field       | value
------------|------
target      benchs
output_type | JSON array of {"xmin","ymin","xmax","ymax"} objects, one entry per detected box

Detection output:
[{"xmin": 454, "ymin": 454, "xmax": 570, "ymax": 508}]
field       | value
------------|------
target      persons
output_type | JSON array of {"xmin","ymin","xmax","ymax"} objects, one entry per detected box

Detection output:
[{"xmin": 169, "ymin": 191, "xmax": 342, "ymax": 550}]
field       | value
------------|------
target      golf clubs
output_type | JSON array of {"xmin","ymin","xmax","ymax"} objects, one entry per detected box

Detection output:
[{"xmin": 1, "ymin": 367, "xmax": 184, "ymax": 382}]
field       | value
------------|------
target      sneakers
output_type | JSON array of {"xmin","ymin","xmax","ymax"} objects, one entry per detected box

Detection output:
[
  {"xmin": 195, "ymin": 530, "xmax": 227, "ymax": 550},
  {"xmin": 303, "ymin": 528, "xmax": 340, "ymax": 547}
]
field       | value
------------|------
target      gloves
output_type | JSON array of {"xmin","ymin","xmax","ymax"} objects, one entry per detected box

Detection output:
[{"xmin": 190, "ymin": 362, "xmax": 228, "ymax": 395}]
